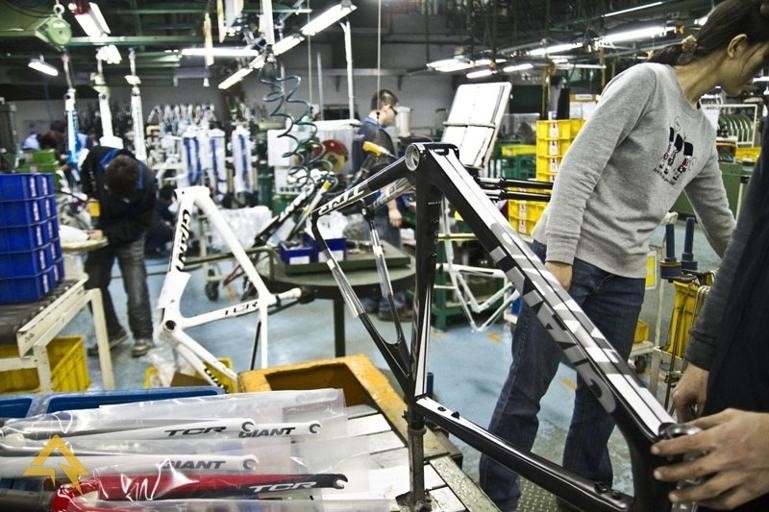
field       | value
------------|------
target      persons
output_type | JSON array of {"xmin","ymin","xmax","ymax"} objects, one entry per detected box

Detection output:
[
  {"xmin": 651, "ymin": 112, "xmax": 769, "ymax": 511},
  {"xmin": 19, "ymin": 131, "xmax": 54, "ymax": 152},
  {"xmin": 141, "ymin": 183, "xmax": 180, "ymax": 258},
  {"xmin": 49, "ymin": 121, "xmax": 100, "ymax": 191},
  {"xmin": 352, "ymin": 90, "xmax": 417, "ymax": 323},
  {"xmin": 66, "ymin": 145, "xmax": 159, "ymax": 358},
  {"xmin": 477, "ymin": 2, "xmax": 769, "ymax": 512}
]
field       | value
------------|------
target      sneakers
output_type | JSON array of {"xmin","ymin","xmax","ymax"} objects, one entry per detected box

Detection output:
[
  {"xmin": 88, "ymin": 329, "xmax": 128, "ymax": 356},
  {"xmin": 131, "ymin": 339, "xmax": 156, "ymax": 357}
]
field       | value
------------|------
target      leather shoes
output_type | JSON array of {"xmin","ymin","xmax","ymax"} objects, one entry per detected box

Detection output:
[{"xmin": 378, "ymin": 308, "xmax": 414, "ymax": 321}]
